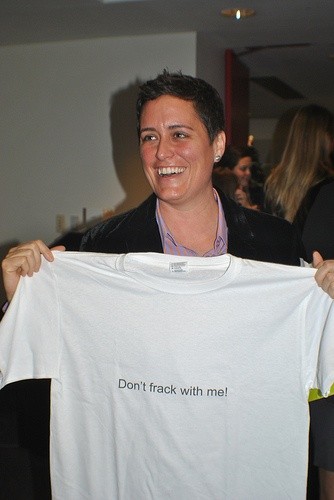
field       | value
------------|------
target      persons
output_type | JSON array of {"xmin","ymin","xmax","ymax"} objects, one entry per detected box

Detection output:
[
  {"xmin": 3, "ymin": 70, "xmax": 334, "ymax": 481},
  {"xmin": 209, "ymin": 129, "xmax": 272, "ymax": 216},
  {"xmin": 263, "ymin": 104, "xmax": 334, "ymax": 272}
]
